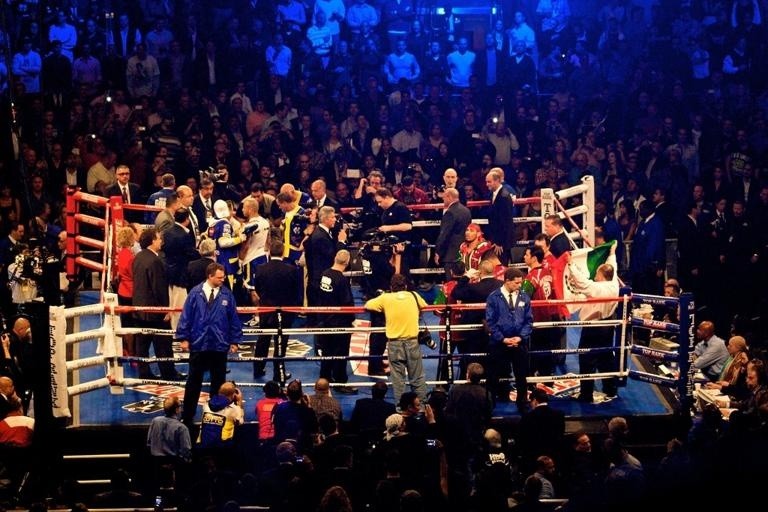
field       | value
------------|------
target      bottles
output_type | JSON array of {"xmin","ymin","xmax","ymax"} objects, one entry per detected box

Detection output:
[{"xmin": 154, "ymin": 494, "xmax": 162, "ymax": 508}]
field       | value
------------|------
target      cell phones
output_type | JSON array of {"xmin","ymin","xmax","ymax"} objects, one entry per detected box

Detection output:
[
  {"xmin": 155, "ymin": 494, "xmax": 162, "ymax": 506},
  {"xmin": 426, "ymin": 439, "xmax": 436, "ymax": 448}
]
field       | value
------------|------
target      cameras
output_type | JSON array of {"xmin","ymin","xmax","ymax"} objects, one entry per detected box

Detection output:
[
  {"xmin": 295, "ymin": 456, "xmax": 304, "ymax": 463},
  {"xmin": 418, "ymin": 330, "xmax": 438, "ymax": 350}
]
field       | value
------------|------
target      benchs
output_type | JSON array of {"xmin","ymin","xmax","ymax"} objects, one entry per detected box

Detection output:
[{"xmin": 48, "ymin": 499, "xmax": 570, "ymax": 512}]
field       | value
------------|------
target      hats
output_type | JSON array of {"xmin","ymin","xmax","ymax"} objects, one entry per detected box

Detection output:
[{"xmin": 213, "ymin": 199, "xmax": 231, "ymax": 219}]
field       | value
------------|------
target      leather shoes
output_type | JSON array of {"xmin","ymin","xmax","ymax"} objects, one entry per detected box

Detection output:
[
  {"xmin": 382, "ymin": 363, "xmax": 389, "ymax": 368},
  {"xmin": 333, "ymin": 386, "xmax": 358, "ymax": 393},
  {"xmin": 163, "ymin": 371, "xmax": 188, "ymax": 381},
  {"xmin": 273, "ymin": 373, "xmax": 292, "ymax": 381},
  {"xmin": 368, "ymin": 369, "xmax": 391, "ymax": 375},
  {"xmin": 139, "ymin": 373, "xmax": 161, "ymax": 379}
]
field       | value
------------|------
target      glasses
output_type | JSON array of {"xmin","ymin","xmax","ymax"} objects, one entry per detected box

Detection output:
[
  {"xmin": 216, "ymin": 274, "xmax": 227, "ymax": 279},
  {"xmin": 117, "ymin": 172, "xmax": 130, "ymax": 176}
]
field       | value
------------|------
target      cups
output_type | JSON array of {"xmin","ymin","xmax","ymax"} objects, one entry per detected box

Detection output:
[
  {"xmin": 294, "ymin": 454, "xmax": 304, "ymax": 462},
  {"xmin": 426, "ymin": 438, "xmax": 436, "ymax": 446},
  {"xmin": 694, "ymin": 383, "xmax": 701, "ymax": 391}
]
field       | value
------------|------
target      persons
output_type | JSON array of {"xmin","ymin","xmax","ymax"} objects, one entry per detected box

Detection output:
[{"xmin": 1, "ymin": 1, "xmax": 767, "ymax": 511}]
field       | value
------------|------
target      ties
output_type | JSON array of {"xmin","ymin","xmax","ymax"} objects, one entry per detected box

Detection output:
[
  {"xmin": 186, "ymin": 207, "xmax": 198, "ymax": 229},
  {"xmin": 205, "ymin": 200, "xmax": 211, "ymax": 210},
  {"xmin": 122, "ymin": 187, "xmax": 128, "ymax": 204},
  {"xmin": 208, "ymin": 288, "xmax": 215, "ymax": 310},
  {"xmin": 318, "ymin": 199, "xmax": 320, "ymax": 207},
  {"xmin": 508, "ymin": 293, "xmax": 513, "ymax": 311}
]
